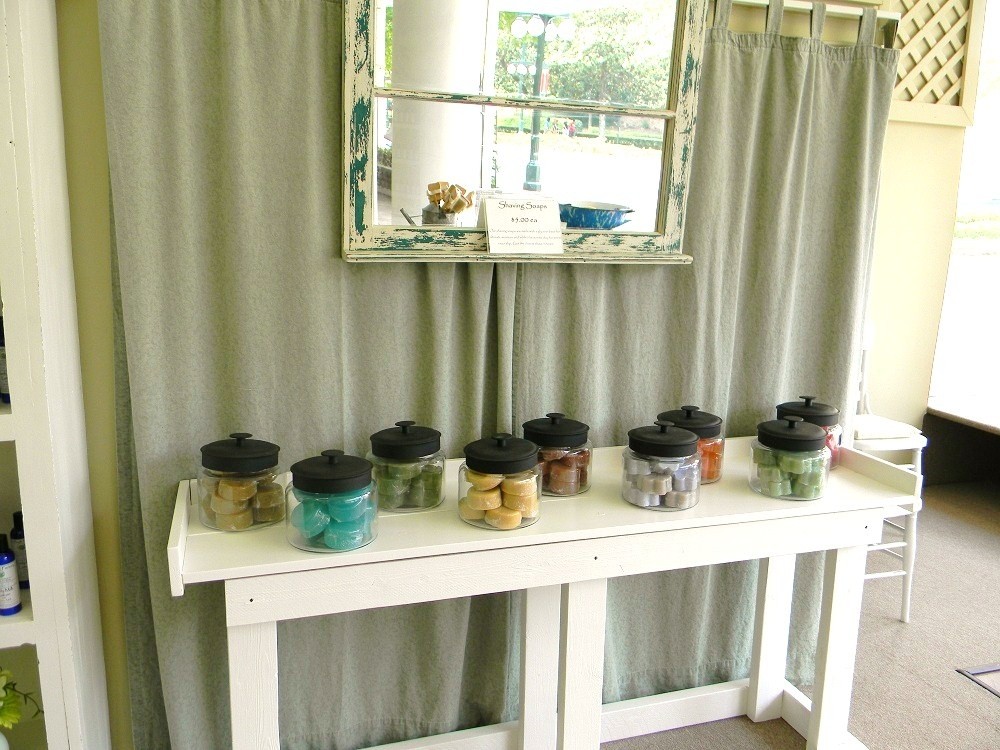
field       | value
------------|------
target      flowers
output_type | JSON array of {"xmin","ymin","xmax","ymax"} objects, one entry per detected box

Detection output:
[{"xmin": 0, "ymin": 668, "xmax": 43, "ymax": 729}]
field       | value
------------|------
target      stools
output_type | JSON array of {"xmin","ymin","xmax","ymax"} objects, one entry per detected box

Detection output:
[{"xmin": 853, "ymin": 395, "xmax": 928, "ymax": 625}]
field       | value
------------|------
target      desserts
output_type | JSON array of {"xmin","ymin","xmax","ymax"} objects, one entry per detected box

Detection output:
[
  {"xmin": 290, "ymin": 481, "xmax": 375, "ymax": 549},
  {"xmin": 697, "ymin": 436, "xmax": 724, "ymax": 482},
  {"xmin": 622, "ymin": 455, "xmax": 698, "ymax": 509},
  {"xmin": 823, "ymin": 426, "xmax": 842, "ymax": 468},
  {"xmin": 200, "ymin": 467, "xmax": 284, "ymax": 531},
  {"xmin": 753, "ymin": 446, "xmax": 828, "ymax": 497},
  {"xmin": 371, "ymin": 457, "xmax": 441, "ymax": 511},
  {"xmin": 460, "ymin": 469, "xmax": 538, "ymax": 530},
  {"xmin": 537, "ymin": 447, "xmax": 590, "ymax": 495}
]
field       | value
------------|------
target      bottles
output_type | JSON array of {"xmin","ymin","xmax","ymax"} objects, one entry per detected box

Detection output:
[
  {"xmin": 748, "ymin": 416, "xmax": 831, "ymax": 501},
  {"xmin": 10, "ymin": 511, "xmax": 30, "ymax": 589},
  {"xmin": 457, "ymin": 432, "xmax": 542, "ymax": 531},
  {"xmin": 776, "ymin": 395, "xmax": 842, "ymax": 470},
  {"xmin": 0, "ymin": 533, "xmax": 22, "ymax": 616},
  {"xmin": 285, "ymin": 449, "xmax": 378, "ymax": 554},
  {"xmin": 366, "ymin": 421, "xmax": 446, "ymax": 514},
  {"xmin": 197, "ymin": 433, "xmax": 286, "ymax": 532},
  {"xmin": 522, "ymin": 413, "xmax": 593, "ymax": 497},
  {"xmin": 622, "ymin": 421, "xmax": 703, "ymax": 512},
  {"xmin": 657, "ymin": 406, "xmax": 724, "ymax": 484}
]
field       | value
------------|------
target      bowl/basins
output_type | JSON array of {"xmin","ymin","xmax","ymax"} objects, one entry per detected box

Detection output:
[{"xmin": 558, "ymin": 199, "xmax": 633, "ymax": 230}]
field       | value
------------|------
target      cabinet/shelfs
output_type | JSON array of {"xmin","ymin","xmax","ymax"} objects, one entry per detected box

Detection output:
[{"xmin": 0, "ymin": 404, "xmax": 110, "ymax": 750}]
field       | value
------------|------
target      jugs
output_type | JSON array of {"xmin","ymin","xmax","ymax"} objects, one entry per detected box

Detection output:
[{"xmin": 400, "ymin": 201, "xmax": 458, "ymax": 226}]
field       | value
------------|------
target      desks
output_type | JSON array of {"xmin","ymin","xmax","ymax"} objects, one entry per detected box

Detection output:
[{"xmin": 166, "ymin": 441, "xmax": 925, "ymax": 750}]
